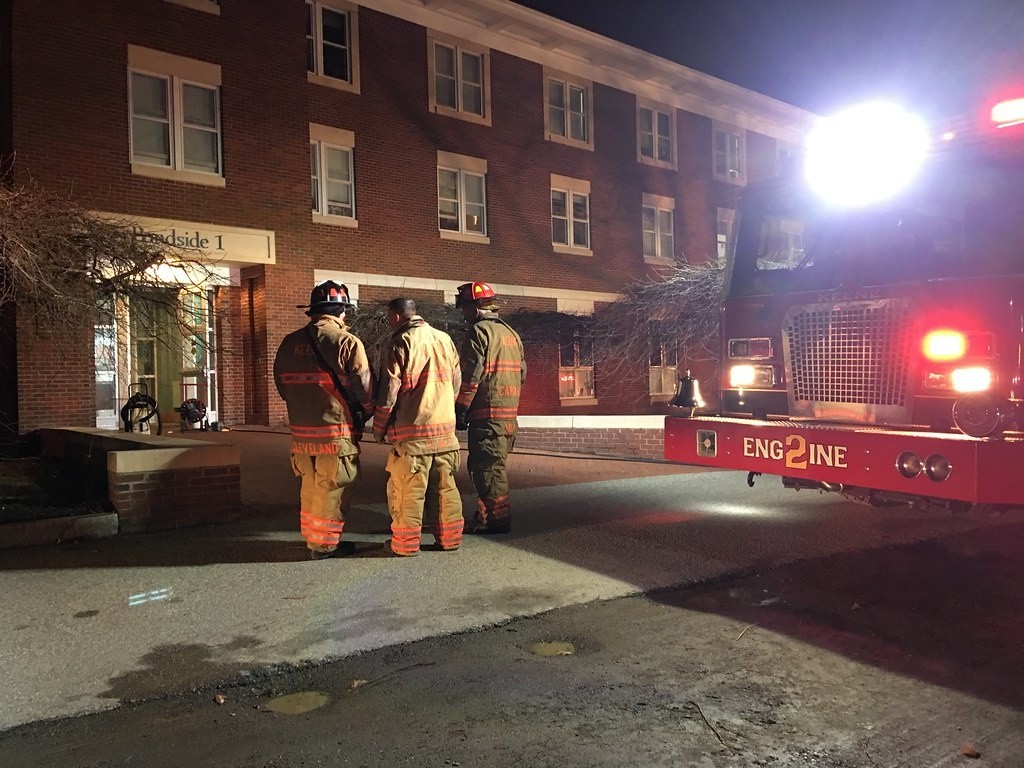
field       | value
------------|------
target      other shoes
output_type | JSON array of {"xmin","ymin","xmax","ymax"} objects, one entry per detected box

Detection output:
[
  {"xmin": 383, "ymin": 540, "xmax": 417, "ymax": 556},
  {"xmin": 312, "ymin": 541, "xmax": 355, "ymax": 559},
  {"xmin": 469, "ymin": 517, "xmax": 512, "ymax": 533}
]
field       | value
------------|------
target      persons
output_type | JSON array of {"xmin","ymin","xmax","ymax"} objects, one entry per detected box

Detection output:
[
  {"xmin": 454, "ymin": 281, "xmax": 527, "ymax": 535},
  {"xmin": 273, "ymin": 279, "xmax": 376, "ymax": 555},
  {"xmin": 372, "ymin": 297, "xmax": 465, "ymax": 555}
]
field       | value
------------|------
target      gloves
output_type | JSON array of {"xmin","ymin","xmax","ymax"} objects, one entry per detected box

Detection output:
[{"xmin": 455, "ymin": 403, "xmax": 468, "ymax": 430}]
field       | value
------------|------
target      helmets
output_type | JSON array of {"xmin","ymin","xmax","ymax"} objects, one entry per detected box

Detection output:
[
  {"xmin": 454, "ymin": 281, "xmax": 499, "ymax": 309},
  {"xmin": 306, "ymin": 278, "xmax": 357, "ymax": 310}
]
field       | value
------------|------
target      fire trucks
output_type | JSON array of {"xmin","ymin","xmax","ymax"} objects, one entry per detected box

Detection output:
[{"xmin": 663, "ymin": 48, "xmax": 1024, "ymax": 520}]
